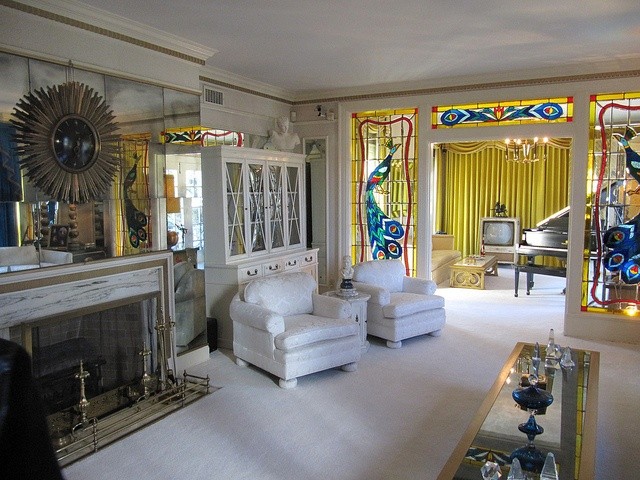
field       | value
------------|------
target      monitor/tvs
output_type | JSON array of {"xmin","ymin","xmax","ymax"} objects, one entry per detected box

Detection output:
[{"xmin": 480, "ymin": 218, "xmax": 520, "ymax": 253}]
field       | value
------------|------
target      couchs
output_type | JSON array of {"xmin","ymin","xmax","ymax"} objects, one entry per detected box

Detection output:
[
  {"xmin": 173, "ymin": 261, "xmax": 204, "ymax": 355},
  {"xmin": 432, "ymin": 235, "xmax": 462, "ymax": 286},
  {"xmin": 352, "ymin": 259, "xmax": 446, "ymax": 349},
  {"xmin": 228, "ymin": 271, "xmax": 363, "ymax": 391},
  {"xmin": 0, "ymin": 246, "xmax": 73, "ymax": 275}
]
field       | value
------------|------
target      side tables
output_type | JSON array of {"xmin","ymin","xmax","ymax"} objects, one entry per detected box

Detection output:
[{"xmin": 322, "ymin": 289, "xmax": 371, "ymax": 355}]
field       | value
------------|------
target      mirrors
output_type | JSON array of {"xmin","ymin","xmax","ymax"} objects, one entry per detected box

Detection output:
[{"xmin": 0, "ymin": 50, "xmax": 212, "ymax": 370}]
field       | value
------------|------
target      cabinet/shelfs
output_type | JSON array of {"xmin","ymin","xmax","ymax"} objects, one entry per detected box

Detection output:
[
  {"xmin": 200, "ymin": 144, "xmax": 320, "ymax": 351},
  {"xmin": 172, "ymin": 244, "xmax": 199, "ymax": 269}
]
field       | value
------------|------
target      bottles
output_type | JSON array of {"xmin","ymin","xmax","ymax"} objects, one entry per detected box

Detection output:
[{"xmin": 480, "ymin": 238, "xmax": 485, "ymax": 256}]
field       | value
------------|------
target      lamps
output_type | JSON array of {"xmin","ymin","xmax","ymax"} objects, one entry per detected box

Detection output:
[{"xmin": 503, "ymin": 137, "xmax": 550, "ymax": 165}]
